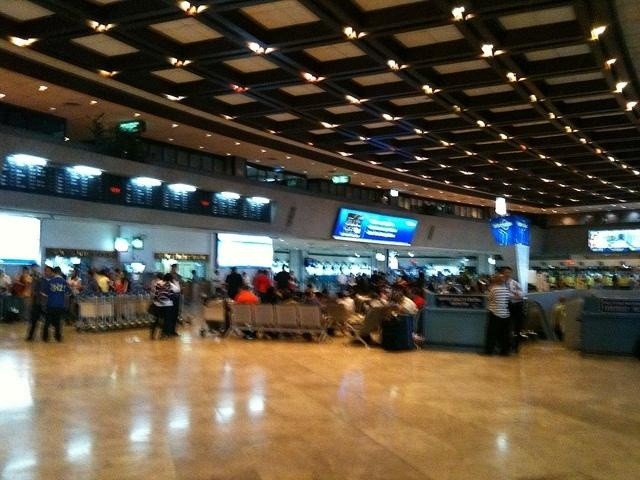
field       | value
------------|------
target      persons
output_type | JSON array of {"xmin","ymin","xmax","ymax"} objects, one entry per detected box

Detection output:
[
  {"xmin": 1, "ymin": 260, "xmax": 198, "ymax": 343},
  {"xmin": 484, "ymin": 266, "xmax": 525, "ymax": 356},
  {"xmin": 529, "ymin": 264, "xmax": 639, "ymax": 289},
  {"xmin": 211, "ymin": 267, "xmax": 492, "ymax": 335}
]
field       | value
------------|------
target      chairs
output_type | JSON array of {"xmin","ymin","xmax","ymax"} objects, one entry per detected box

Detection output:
[
  {"xmin": 226, "ymin": 300, "xmax": 334, "ymax": 344},
  {"xmin": 344, "ymin": 303, "xmax": 399, "ymax": 349}
]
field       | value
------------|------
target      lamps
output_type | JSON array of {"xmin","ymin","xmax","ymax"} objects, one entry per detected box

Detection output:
[
  {"xmin": 114, "ymin": 237, "xmax": 129, "ymax": 253},
  {"xmin": 131, "ymin": 234, "xmax": 145, "ymax": 249},
  {"xmin": 132, "ymin": 254, "xmax": 146, "ymax": 274}
]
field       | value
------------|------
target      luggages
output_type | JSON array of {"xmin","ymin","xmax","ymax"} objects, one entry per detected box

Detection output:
[{"xmin": 380, "ymin": 313, "xmax": 414, "ymax": 351}]
